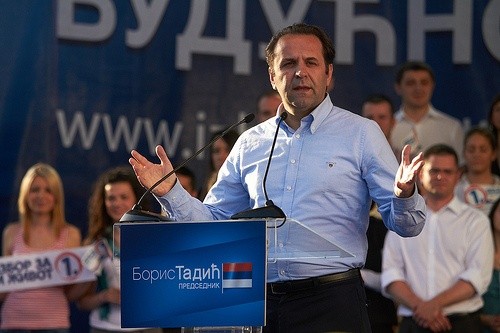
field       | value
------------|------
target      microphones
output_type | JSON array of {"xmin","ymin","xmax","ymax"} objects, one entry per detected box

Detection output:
[
  {"xmin": 118, "ymin": 113, "xmax": 255, "ymax": 222},
  {"xmin": 229, "ymin": 112, "xmax": 288, "ymax": 218}
]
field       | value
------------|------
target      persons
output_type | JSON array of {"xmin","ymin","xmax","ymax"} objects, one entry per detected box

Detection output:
[
  {"xmin": 130, "ymin": 23, "xmax": 428, "ymax": 333},
  {"xmin": 0, "ymin": 64, "xmax": 500, "ymax": 333}
]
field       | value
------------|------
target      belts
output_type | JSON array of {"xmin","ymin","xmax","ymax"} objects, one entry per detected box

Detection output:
[{"xmin": 267, "ymin": 268, "xmax": 361, "ymax": 295}]
